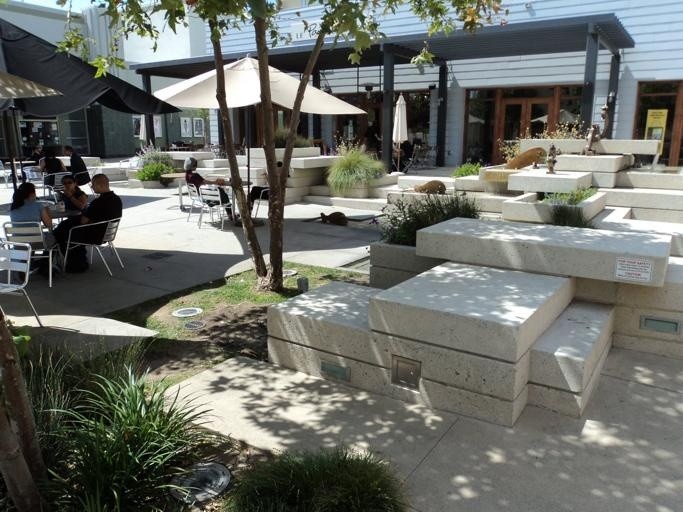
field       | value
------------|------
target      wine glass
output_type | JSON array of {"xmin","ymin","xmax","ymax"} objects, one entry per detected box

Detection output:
[{"xmin": 545, "ymin": 159, "xmax": 556, "ymax": 174}]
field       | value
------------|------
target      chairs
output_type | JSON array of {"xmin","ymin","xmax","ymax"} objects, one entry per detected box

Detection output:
[
  {"xmin": 370, "ymin": 143, "xmax": 436, "ymax": 174},
  {"xmin": 183, "ymin": 181, "xmax": 295, "ymax": 230},
  {"xmin": 210, "ymin": 137, "xmax": 246, "ymax": 159},
  {"xmin": 0, "ymin": 159, "xmax": 127, "ymax": 327}
]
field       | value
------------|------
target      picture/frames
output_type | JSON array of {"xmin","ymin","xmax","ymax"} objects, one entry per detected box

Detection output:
[
  {"xmin": 132, "ymin": 116, "xmax": 141, "ymax": 138},
  {"xmin": 180, "ymin": 117, "xmax": 193, "ymax": 137},
  {"xmin": 193, "ymin": 118, "xmax": 205, "ymax": 137},
  {"xmin": 154, "ymin": 116, "xmax": 163, "ymax": 137}
]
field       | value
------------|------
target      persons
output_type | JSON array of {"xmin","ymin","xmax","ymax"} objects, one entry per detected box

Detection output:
[
  {"xmin": 183, "ymin": 156, "xmax": 238, "ymax": 221},
  {"xmin": 234, "ymin": 160, "xmax": 291, "ymax": 227},
  {"xmin": 9, "ymin": 183, "xmax": 53, "ymax": 281},
  {"xmin": 53, "ymin": 176, "xmax": 86, "ymax": 229},
  {"xmin": 365, "ymin": 120, "xmax": 380, "ymax": 152},
  {"xmin": 20, "ymin": 146, "xmax": 41, "ymax": 179},
  {"xmin": 62, "ymin": 145, "xmax": 90, "ymax": 186},
  {"xmin": 41, "ymin": 145, "xmax": 68, "ymax": 175},
  {"xmin": 53, "ymin": 175, "xmax": 120, "ymax": 274},
  {"xmin": 395, "ymin": 141, "xmax": 412, "ymax": 173}
]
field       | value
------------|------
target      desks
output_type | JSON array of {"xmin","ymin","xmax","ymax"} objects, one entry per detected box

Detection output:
[
  {"xmin": 173, "ymin": 142, "xmax": 184, "ymax": 151},
  {"xmin": 160, "ymin": 173, "xmax": 185, "ymax": 209},
  {"xmin": 170, "ymin": 147, "xmax": 191, "ymax": 152}
]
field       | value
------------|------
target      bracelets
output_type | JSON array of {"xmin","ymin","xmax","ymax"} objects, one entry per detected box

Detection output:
[{"xmin": 68, "ymin": 195, "xmax": 72, "ymax": 199}]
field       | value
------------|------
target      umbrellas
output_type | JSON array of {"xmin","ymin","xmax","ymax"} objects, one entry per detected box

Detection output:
[
  {"xmin": 150, "ymin": 55, "xmax": 371, "ymax": 191},
  {"xmin": 392, "ymin": 92, "xmax": 409, "ymax": 170}
]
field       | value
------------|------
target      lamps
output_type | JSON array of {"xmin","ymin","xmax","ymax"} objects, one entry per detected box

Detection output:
[{"xmin": 359, "ymin": 81, "xmax": 380, "ymax": 100}]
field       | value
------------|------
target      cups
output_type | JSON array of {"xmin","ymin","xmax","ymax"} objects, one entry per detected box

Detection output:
[{"xmin": 56, "ymin": 201, "xmax": 65, "ymax": 212}]
